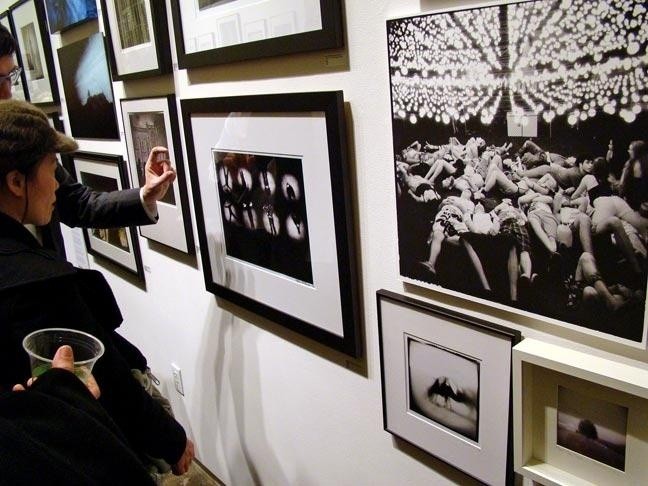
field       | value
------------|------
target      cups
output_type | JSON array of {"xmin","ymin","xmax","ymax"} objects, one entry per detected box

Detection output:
[{"xmin": 23, "ymin": 327, "xmax": 107, "ymax": 386}]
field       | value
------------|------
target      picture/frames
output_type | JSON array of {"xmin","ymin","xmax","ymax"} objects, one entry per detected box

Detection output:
[
  {"xmin": 120, "ymin": 94, "xmax": 197, "ymax": 254},
  {"xmin": 511, "ymin": 337, "xmax": 648, "ymax": 485},
  {"xmin": 44, "ymin": 1, "xmax": 99, "ymax": 34},
  {"xmin": 386, "ymin": 0, "xmax": 647, "ymax": 351},
  {"xmin": 170, "ymin": 1, "xmax": 346, "ymax": 70},
  {"xmin": 0, "ymin": 10, "xmax": 31, "ymax": 102},
  {"xmin": 100, "ymin": 0, "xmax": 173, "ymax": 82},
  {"xmin": 44, "ymin": 111, "xmax": 73, "ymax": 175},
  {"xmin": 56, "ymin": 32, "xmax": 120, "ymax": 141},
  {"xmin": 8, "ymin": 0, "xmax": 60, "ymax": 105},
  {"xmin": 180, "ymin": 89, "xmax": 367, "ymax": 362},
  {"xmin": 376, "ymin": 289, "xmax": 521, "ymax": 485},
  {"xmin": 68, "ymin": 150, "xmax": 144, "ymax": 277}
]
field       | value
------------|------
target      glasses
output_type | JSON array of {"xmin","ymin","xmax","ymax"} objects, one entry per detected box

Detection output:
[{"xmin": 0, "ymin": 65, "xmax": 21, "ymax": 84}]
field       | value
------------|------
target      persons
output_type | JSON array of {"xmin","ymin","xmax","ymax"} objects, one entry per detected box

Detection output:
[
  {"xmin": 396, "ymin": 137, "xmax": 647, "ymax": 313},
  {"xmin": 0, "ymin": 21, "xmax": 176, "ymax": 258},
  {"xmin": 1, "ymin": 344, "xmax": 156, "ymax": 485},
  {"xmin": 0, "ymin": 99, "xmax": 195, "ymax": 476}
]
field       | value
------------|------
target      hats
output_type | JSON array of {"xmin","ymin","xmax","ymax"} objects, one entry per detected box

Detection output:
[{"xmin": 0, "ymin": 99, "xmax": 78, "ymax": 165}]
